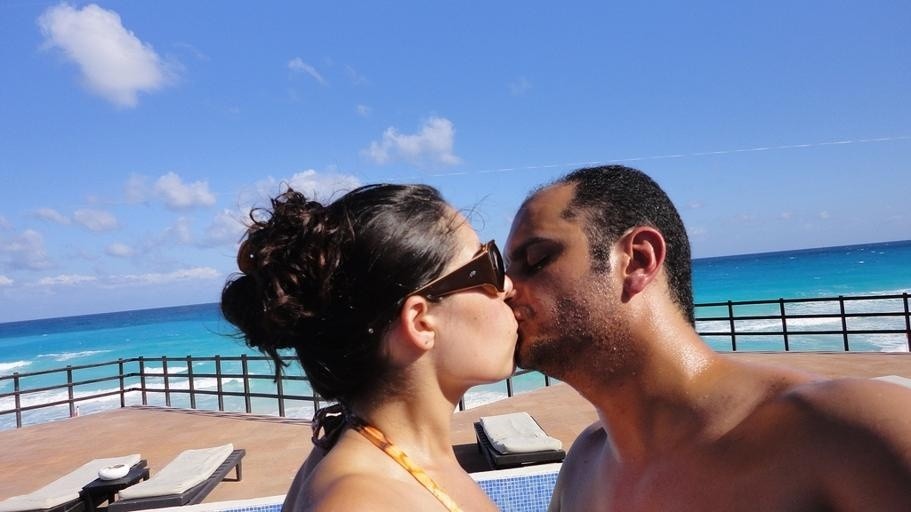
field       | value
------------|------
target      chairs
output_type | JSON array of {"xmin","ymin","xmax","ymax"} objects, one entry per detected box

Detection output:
[
  {"xmin": 0, "ymin": 454, "xmax": 147, "ymax": 512},
  {"xmin": 107, "ymin": 442, "xmax": 246, "ymax": 512},
  {"xmin": 473, "ymin": 414, "xmax": 566, "ymax": 469}
]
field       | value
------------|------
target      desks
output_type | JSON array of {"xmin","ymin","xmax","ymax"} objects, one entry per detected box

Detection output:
[{"xmin": 82, "ymin": 466, "xmax": 149, "ymax": 512}]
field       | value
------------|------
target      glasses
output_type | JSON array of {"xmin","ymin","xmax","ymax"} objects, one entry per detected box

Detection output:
[{"xmin": 363, "ymin": 240, "xmax": 503, "ymax": 349}]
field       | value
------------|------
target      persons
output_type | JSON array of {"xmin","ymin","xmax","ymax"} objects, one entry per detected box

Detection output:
[
  {"xmin": 501, "ymin": 159, "xmax": 910, "ymax": 511},
  {"xmin": 202, "ymin": 175, "xmax": 526, "ymax": 510}
]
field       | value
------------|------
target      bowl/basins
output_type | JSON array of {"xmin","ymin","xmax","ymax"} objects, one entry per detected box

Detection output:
[{"xmin": 96, "ymin": 463, "xmax": 130, "ymax": 481}]
